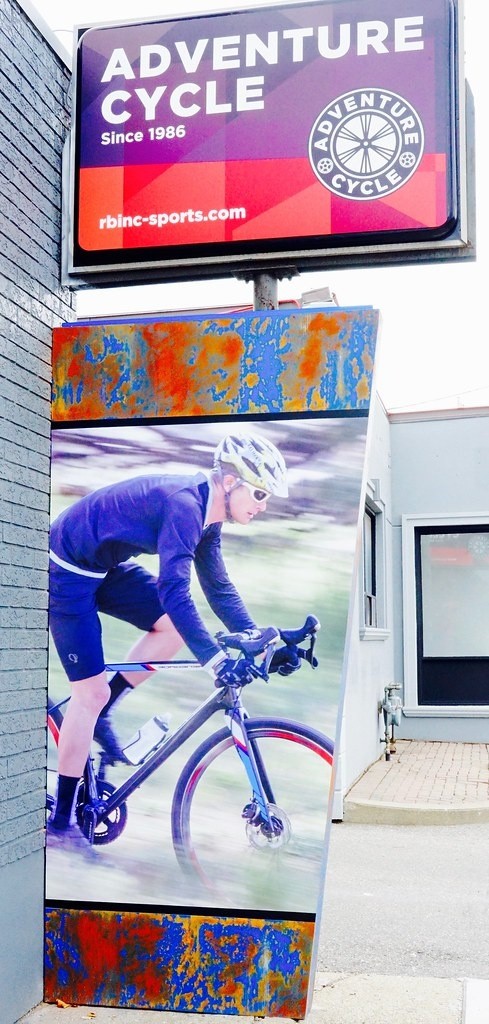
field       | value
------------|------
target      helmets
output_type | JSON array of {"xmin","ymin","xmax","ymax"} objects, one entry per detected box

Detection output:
[{"xmin": 213, "ymin": 433, "xmax": 289, "ymax": 498}]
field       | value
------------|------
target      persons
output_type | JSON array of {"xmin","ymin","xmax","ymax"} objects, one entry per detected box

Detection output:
[{"xmin": 45, "ymin": 432, "xmax": 303, "ymax": 862}]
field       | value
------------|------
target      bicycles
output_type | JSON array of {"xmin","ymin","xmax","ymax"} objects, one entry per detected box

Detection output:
[{"xmin": 47, "ymin": 617, "xmax": 336, "ymax": 913}]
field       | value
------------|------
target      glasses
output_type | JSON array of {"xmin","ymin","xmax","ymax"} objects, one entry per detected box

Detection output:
[{"xmin": 236, "ymin": 478, "xmax": 271, "ymax": 503}]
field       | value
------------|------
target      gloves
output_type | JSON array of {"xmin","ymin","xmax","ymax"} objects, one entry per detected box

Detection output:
[
  {"xmin": 269, "ymin": 645, "xmax": 319, "ymax": 676},
  {"xmin": 214, "ymin": 658, "xmax": 270, "ymax": 688}
]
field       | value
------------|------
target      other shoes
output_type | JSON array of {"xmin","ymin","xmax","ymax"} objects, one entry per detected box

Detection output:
[
  {"xmin": 93, "ymin": 716, "xmax": 131, "ymax": 765},
  {"xmin": 47, "ymin": 815, "xmax": 102, "ymax": 862}
]
